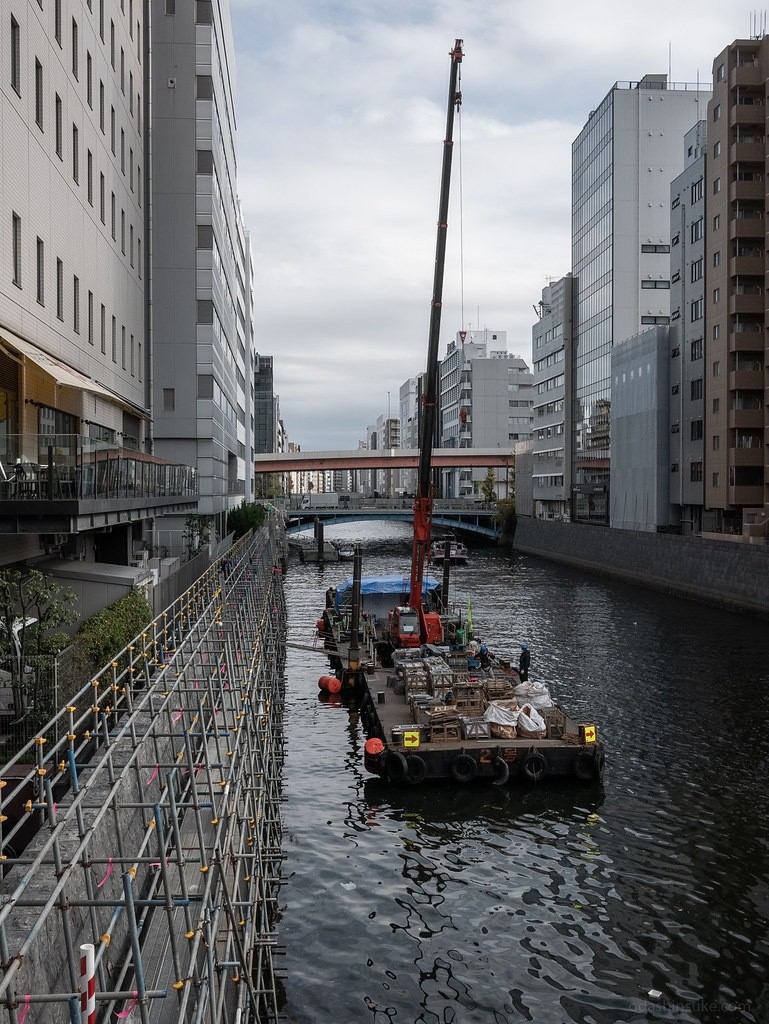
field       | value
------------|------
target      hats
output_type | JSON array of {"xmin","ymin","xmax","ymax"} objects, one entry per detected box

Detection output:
[
  {"xmin": 480, "ymin": 646, "xmax": 488, "ymax": 654},
  {"xmin": 520, "ymin": 643, "xmax": 528, "ymax": 650}
]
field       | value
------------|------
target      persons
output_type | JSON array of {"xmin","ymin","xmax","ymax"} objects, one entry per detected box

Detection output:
[
  {"xmin": 474, "ymin": 645, "xmax": 495, "ymax": 680},
  {"xmin": 519, "ymin": 643, "xmax": 530, "ymax": 684},
  {"xmin": 221, "ymin": 554, "xmax": 233, "ymax": 585}
]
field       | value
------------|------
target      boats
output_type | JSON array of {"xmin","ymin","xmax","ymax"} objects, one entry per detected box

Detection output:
[
  {"xmin": 339, "ymin": 549, "xmax": 355, "ymax": 561},
  {"xmin": 322, "ymin": 573, "xmax": 601, "ymax": 784},
  {"xmin": 430, "ymin": 539, "xmax": 469, "ymax": 566}
]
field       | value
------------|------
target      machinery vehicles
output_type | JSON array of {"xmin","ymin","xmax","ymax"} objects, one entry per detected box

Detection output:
[{"xmin": 378, "ymin": 38, "xmax": 469, "ymax": 670}]
